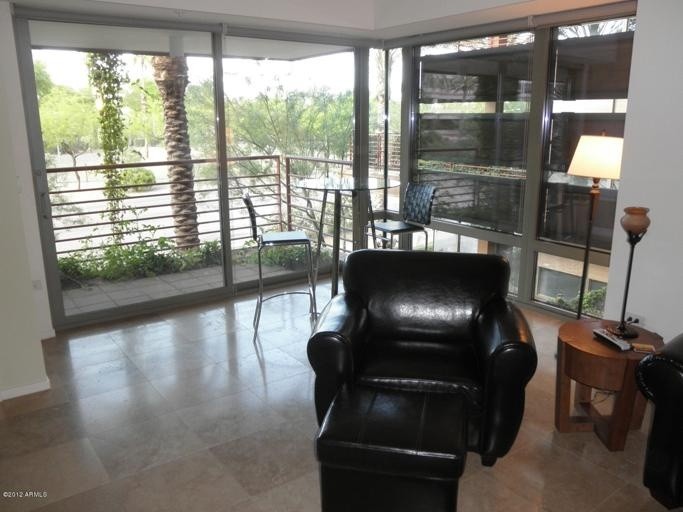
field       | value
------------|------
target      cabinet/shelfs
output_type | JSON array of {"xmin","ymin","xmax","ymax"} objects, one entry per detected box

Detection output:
[{"xmin": 286, "ymin": 177, "xmax": 402, "ymax": 314}]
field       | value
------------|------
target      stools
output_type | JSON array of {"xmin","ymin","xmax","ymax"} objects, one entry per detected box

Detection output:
[{"xmin": 312, "ymin": 377, "xmax": 479, "ymax": 510}]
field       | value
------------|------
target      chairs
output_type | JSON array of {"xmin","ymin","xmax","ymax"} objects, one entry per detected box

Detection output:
[
  {"xmin": 632, "ymin": 332, "xmax": 682, "ymax": 512},
  {"xmin": 240, "ymin": 182, "xmax": 537, "ymax": 468}
]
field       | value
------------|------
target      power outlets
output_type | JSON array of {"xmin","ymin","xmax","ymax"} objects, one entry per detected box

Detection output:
[{"xmin": 624, "ymin": 312, "xmax": 646, "ymax": 327}]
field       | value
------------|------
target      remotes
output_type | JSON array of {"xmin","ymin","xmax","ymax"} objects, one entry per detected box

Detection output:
[{"xmin": 592, "ymin": 329, "xmax": 631, "ymax": 352}]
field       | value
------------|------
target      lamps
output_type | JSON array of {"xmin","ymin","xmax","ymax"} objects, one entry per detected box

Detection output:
[{"xmin": 606, "ymin": 205, "xmax": 651, "ymax": 338}]
